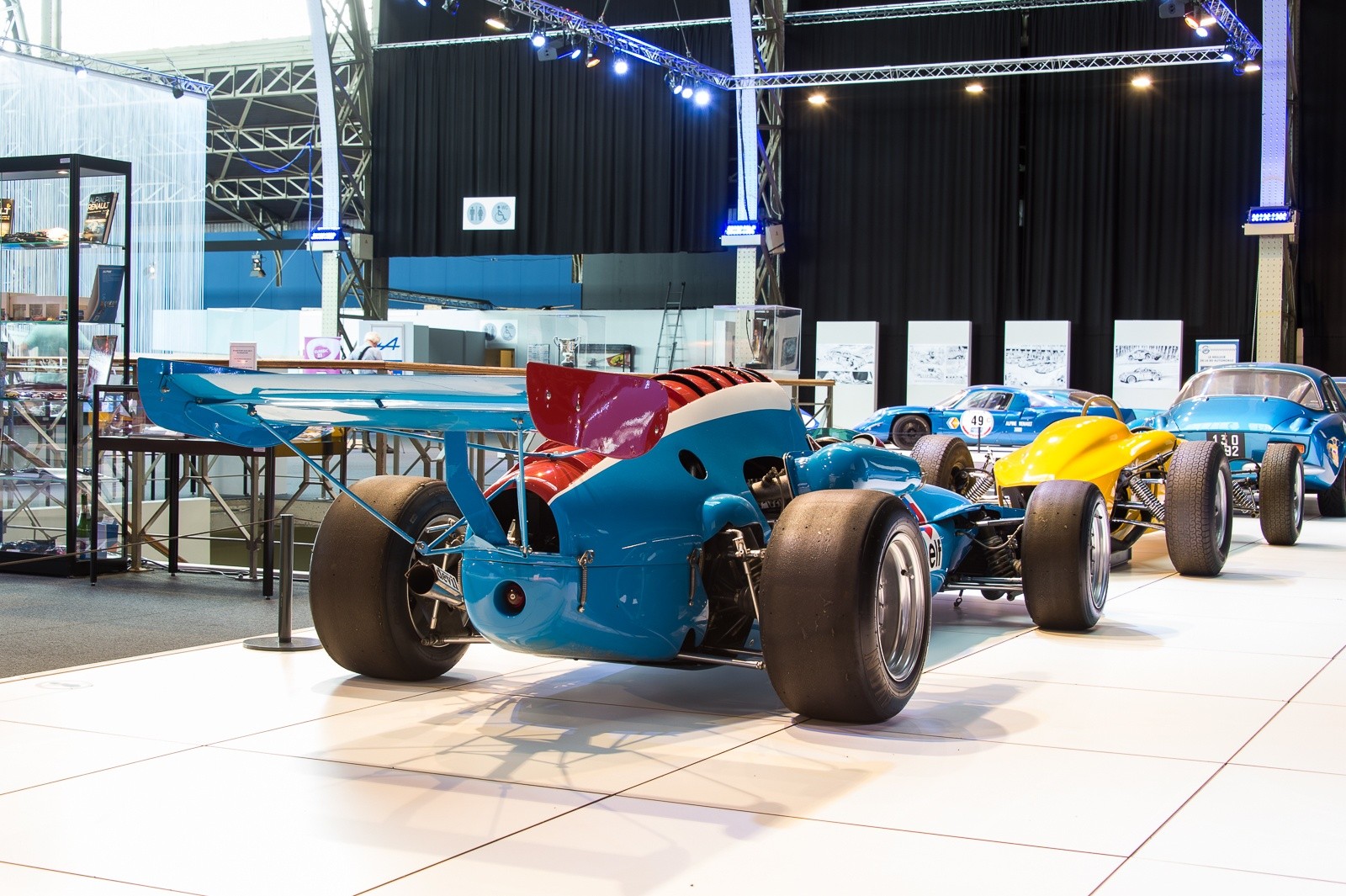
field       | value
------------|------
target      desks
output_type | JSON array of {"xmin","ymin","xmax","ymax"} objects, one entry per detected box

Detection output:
[
  {"xmin": 88, "ymin": 381, "xmax": 348, "ymax": 600},
  {"xmin": 772, "ymin": 378, "xmax": 836, "ymax": 429}
]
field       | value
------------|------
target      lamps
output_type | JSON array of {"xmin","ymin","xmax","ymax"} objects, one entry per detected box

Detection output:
[
  {"xmin": 692, "ymin": 78, "xmax": 710, "ymax": 106},
  {"xmin": 1240, "ymin": 59, "xmax": 1261, "ymax": 73},
  {"xmin": 485, "ymin": 5, "xmax": 520, "ymax": 32},
  {"xmin": 565, "ymin": 31, "xmax": 583, "ymax": 60},
  {"xmin": 530, "ymin": 20, "xmax": 547, "ymax": 48},
  {"xmin": 611, "ymin": 49, "xmax": 628, "ymax": 74},
  {"xmin": 679, "ymin": 75, "xmax": 693, "ymax": 99},
  {"xmin": 1132, "ymin": 67, "xmax": 1151, "ymax": 86},
  {"xmin": 1184, "ymin": 3, "xmax": 1204, "ymax": 29},
  {"xmin": 249, "ymin": 250, "xmax": 267, "ymax": 279},
  {"xmin": 964, "ymin": 76, "xmax": 984, "ymax": 93},
  {"xmin": 1222, "ymin": 37, "xmax": 1237, "ymax": 62},
  {"xmin": 806, "ymin": 84, "xmax": 828, "ymax": 105},
  {"xmin": 441, "ymin": 0, "xmax": 460, "ymax": 16},
  {"xmin": 1196, "ymin": 24, "xmax": 1212, "ymax": 38},
  {"xmin": 417, "ymin": 0, "xmax": 432, "ymax": 7},
  {"xmin": 1200, "ymin": 11, "xmax": 1217, "ymax": 26},
  {"xmin": 584, "ymin": 39, "xmax": 600, "ymax": 68},
  {"xmin": 664, "ymin": 69, "xmax": 683, "ymax": 95}
]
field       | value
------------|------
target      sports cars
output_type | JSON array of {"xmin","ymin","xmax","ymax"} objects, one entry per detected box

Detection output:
[{"xmin": 1150, "ymin": 362, "xmax": 1346, "ymax": 518}]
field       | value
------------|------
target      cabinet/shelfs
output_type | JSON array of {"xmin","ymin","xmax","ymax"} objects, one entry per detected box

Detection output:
[
  {"xmin": 574, "ymin": 344, "xmax": 637, "ymax": 373},
  {"xmin": 0, "ymin": 152, "xmax": 134, "ymax": 580}
]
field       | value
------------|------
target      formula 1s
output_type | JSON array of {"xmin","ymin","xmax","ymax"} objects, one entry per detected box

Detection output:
[
  {"xmin": 796, "ymin": 363, "xmax": 1305, "ymax": 633},
  {"xmin": 309, "ymin": 363, "xmax": 937, "ymax": 729}
]
